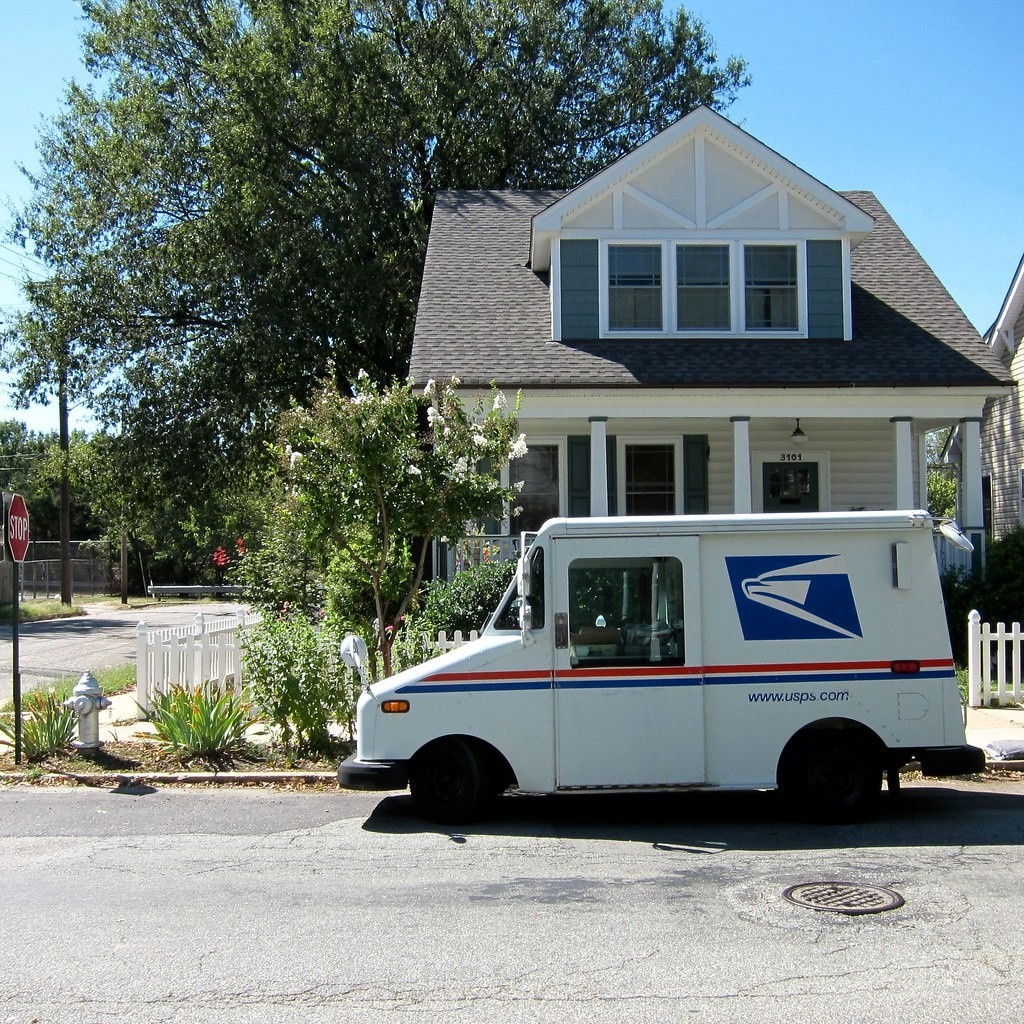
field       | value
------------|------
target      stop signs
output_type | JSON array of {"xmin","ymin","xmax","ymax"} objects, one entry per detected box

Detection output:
[{"xmin": 7, "ymin": 492, "xmax": 31, "ymax": 563}]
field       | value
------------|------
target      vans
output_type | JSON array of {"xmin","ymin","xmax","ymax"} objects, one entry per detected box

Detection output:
[{"xmin": 336, "ymin": 509, "xmax": 987, "ymax": 826}]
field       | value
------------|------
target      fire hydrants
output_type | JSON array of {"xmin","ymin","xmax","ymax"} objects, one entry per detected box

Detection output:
[{"xmin": 64, "ymin": 670, "xmax": 113, "ymax": 757}]
